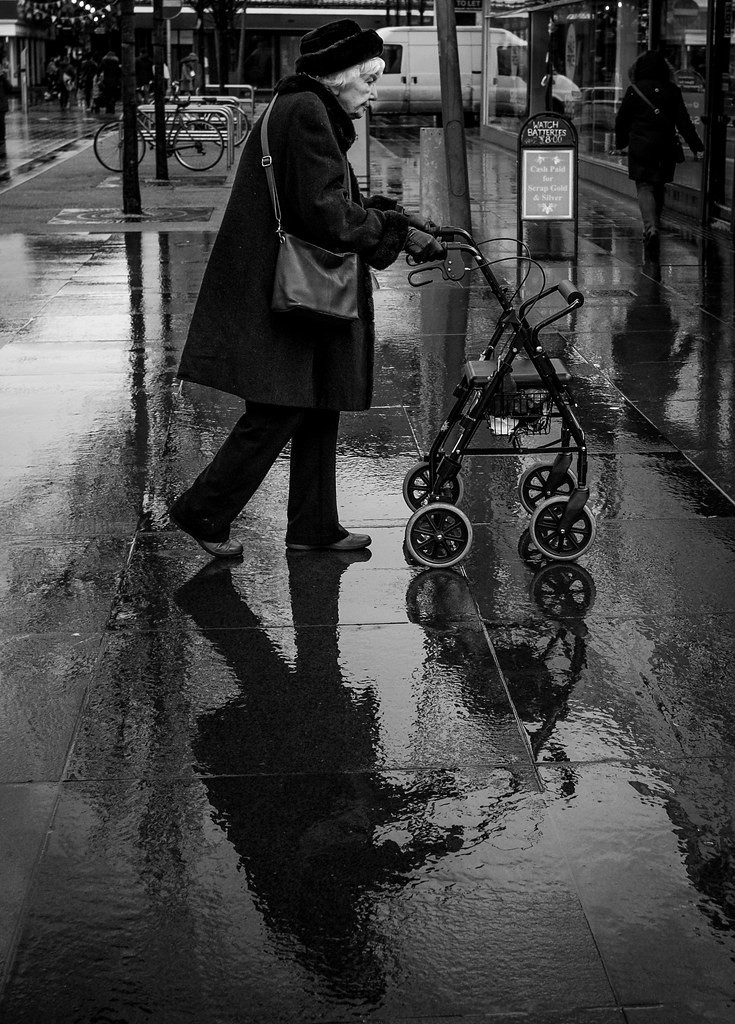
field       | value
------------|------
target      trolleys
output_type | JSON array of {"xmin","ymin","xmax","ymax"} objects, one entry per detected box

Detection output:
[{"xmin": 398, "ymin": 225, "xmax": 596, "ymax": 568}]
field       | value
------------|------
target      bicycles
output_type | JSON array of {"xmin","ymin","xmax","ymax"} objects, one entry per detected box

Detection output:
[{"xmin": 92, "ymin": 78, "xmax": 250, "ymax": 173}]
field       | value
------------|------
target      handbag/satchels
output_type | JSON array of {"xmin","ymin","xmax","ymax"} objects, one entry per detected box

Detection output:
[
  {"xmin": 274, "ymin": 229, "xmax": 359, "ymax": 322},
  {"xmin": 673, "ymin": 136, "xmax": 685, "ymax": 164}
]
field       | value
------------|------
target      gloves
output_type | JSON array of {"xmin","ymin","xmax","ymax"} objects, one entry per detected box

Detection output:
[
  {"xmin": 697, "ymin": 150, "xmax": 705, "ymax": 158},
  {"xmin": 406, "ymin": 229, "xmax": 448, "ymax": 263},
  {"xmin": 616, "ymin": 147, "xmax": 625, "ymax": 154},
  {"xmin": 401, "ymin": 208, "xmax": 439, "ymax": 239}
]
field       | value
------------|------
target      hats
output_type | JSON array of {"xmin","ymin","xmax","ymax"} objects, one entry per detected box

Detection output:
[
  {"xmin": 180, "ymin": 53, "xmax": 199, "ymax": 62},
  {"xmin": 295, "ymin": 19, "xmax": 383, "ymax": 77}
]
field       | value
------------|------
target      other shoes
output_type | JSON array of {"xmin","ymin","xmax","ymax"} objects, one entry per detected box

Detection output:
[
  {"xmin": 645, "ymin": 226, "xmax": 661, "ymax": 251},
  {"xmin": 287, "ymin": 532, "xmax": 372, "ymax": 548},
  {"xmin": 168, "ymin": 512, "xmax": 243, "ymax": 555}
]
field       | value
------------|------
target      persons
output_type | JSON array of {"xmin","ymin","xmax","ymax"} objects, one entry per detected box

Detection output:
[
  {"xmin": 168, "ymin": 18, "xmax": 446, "ymax": 557},
  {"xmin": 45, "ymin": 45, "xmax": 170, "ymax": 115},
  {"xmin": 0, "ymin": 66, "xmax": 10, "ymax": 146},
  {"xmin": 180, "ymin": 53, "xmax": 202, "ymax": 96},
  {"xmin": 615, "ymin": 50, "xmax": 704, "ymax": 250}
]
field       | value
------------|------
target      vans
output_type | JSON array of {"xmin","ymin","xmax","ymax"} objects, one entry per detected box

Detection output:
[{"xmin": 366, "ymin": 25, "xmax": 584, "ymax": 123}]
